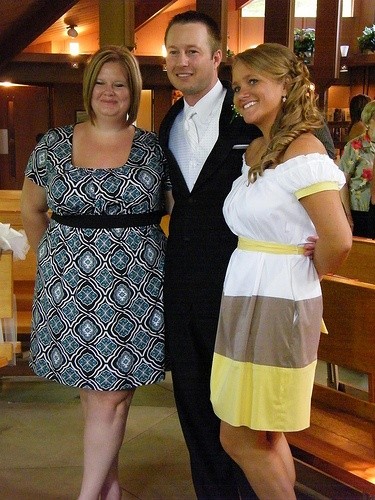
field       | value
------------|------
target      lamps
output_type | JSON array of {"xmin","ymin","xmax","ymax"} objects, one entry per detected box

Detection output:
[
  {"xmin": 71, "ymin": 62, "xmax": 79, "ymax": 69},
  {"xmin": 340, "ymin": 44, "xmax": 349, "ymax": 72},
  {"xmin": 162, "ymin": 64, "xmax": 167, "ymax": 72},
  {"xmin": 66, "ymin": 24, "xmax": 79, "ymax": 38}
]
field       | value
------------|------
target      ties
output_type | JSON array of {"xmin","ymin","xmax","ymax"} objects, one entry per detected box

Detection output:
[{"xmin": 183, "ymin": 110, "xmax": 199, "ymax": 152}]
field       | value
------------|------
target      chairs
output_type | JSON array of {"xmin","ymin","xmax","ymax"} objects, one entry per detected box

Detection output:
[{"xmin": 326, "ymin": 235, "xmax": 375, "ymax": 404}]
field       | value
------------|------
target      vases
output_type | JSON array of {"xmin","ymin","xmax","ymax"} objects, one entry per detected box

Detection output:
[{"xmin": 294, "ymin": 45, "xmax": 313, "ymax": 64}]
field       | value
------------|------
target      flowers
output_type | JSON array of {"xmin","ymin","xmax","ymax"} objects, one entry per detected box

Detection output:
[
  {"xmin": 294, "ymin": 28, "xmax": 316, "ymax": 52},
  {"xmin": 229, "ymin": 104, "xmax": 244, "ymax": 124},
  {"xmin": 356, "ymin": 23, "xmax": 375, "ymax": 53}
]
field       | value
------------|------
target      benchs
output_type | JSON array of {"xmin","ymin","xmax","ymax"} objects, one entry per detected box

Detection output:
[
  {"xmin": 281, "ymin": 274, "xmax": 375, "ymax": 500},
  {"xmin": 0, "ymin": 190, "xmax": 53, "ymax": 371}
]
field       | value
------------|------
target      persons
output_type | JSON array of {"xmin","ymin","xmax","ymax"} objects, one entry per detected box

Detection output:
[
  {"xmin": 19, "ymin": 45, "xmax": 176, "ymax": 500},
  {"xmin": 338, "ymin": 99, "xmax": 375, "ymax": 240},
  {"xmin": 207, "ymin": 40, "xmax": 354, "ymax": 500},
  {"xmin": 156, "ymin": 8, "xmax": 318, "ymax": 500},
  {"xmin": 346, "ymin": 94, "xmax": 372, "ymax": 140}
]
